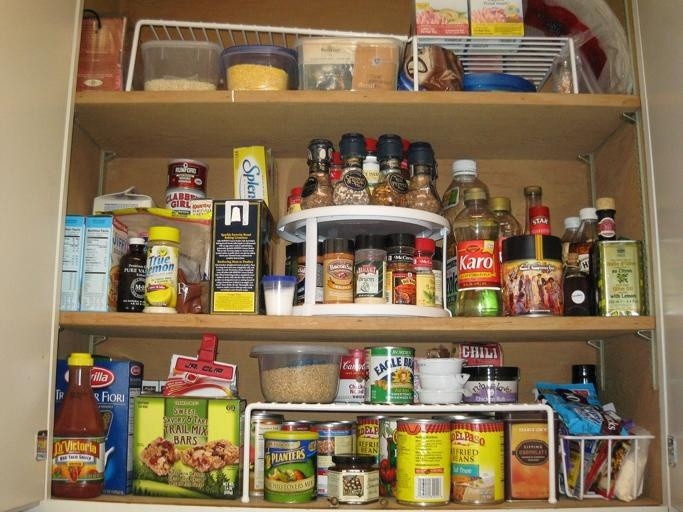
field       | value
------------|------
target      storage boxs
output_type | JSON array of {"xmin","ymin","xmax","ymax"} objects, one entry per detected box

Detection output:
[{"xmin": 292, "ymin": 36, "xmax": 406, "ymax": 90}]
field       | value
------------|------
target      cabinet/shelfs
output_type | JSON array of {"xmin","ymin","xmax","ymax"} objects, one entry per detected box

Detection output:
[{"xmin": 43, "ymin": 0, "xmax": 682, "ymax": 510}]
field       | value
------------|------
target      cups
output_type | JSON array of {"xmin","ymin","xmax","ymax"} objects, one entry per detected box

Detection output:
[{"xmin": 261, "ymin": 275, "xmax": 295, "ymax": 315}]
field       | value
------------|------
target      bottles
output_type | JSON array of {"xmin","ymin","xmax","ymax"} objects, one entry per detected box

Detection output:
[
  {"xmin": 571, "ymin": 364, "xmax": 597, "ymax": 392},
  {"xmin": 274, "ymin": 234, "xmax": 441, "ymax": 309},
  {"xmin": 441, "ymin": 157, "xmax": 623, "ymax": 320},
  {"xmin": 49, "ymin": 350, "xmax": 107, "ymax": 498},
  {"xmin": 118, "ymin": 236, "xmax": 145, "ymax": 311},
  {"xmin": 141, "ymin": 225, "xmax": 182, "ymax": 316},
  {"xmin": 282, "ymin": 132, "xmax": 441, "ymax": 212}
]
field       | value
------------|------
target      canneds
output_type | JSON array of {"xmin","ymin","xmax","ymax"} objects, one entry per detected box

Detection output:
[
  {"xmin": 311, "ymin": 420, "xmax": 354, "ymax": 500},
  {"xmin": 240, "ymin": 409, "xmax": 284, "ymax": 498},
  {"xmin": 284, "ymin": 419, "xmax": 311, "ymax": 432},
  {"xmin": 333, "ymin": 349, "xmax": 365, "ymax": 403},
  {"xmin": 167, "ymin": 158, "xmax": 208, "ymax": 193},
  {"xmin": 380, "ymin": 416, "xmax": 398, "ymax": 498},
  {"xmin": 453, "ymin": 412, "xmax": 506, "ymax": 506},
  {"xmin": 395, "ymin": 418, "xmax": 453, "ymax": 508},
  {"xmin": 163, "ymin": 189, "xmax": 206, "ymax": 215},
  {"xmin": 355, "ymin": 416, "xmax": 380, "ymax": 455},
  {"xmin": 364, "ymin": 346, "xmax": 415, "ymax": 405}
]
